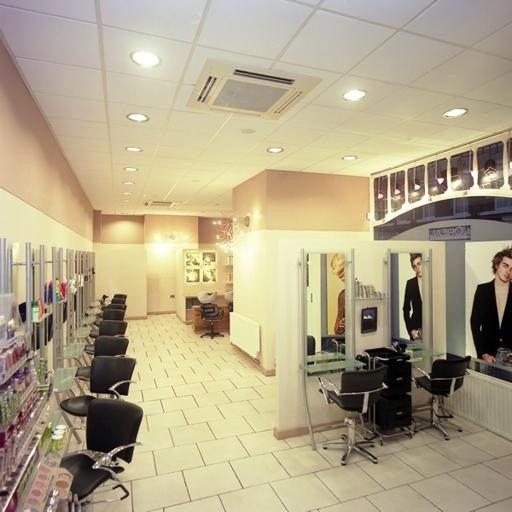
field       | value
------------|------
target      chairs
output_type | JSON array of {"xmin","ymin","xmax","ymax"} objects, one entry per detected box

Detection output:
[
  {"xmin": 59, "ymin": 399, "xmax": 143, "ymax": 512},
  {"xmin": 307, "ymin": 335, "xmax": 315, "ymax": 365},
  {"xmin": 318, "ymin": 366, "xmax": 388, "ymax": 466},
  {"xmin": 414, "ymin": 356, "xmax": 472, "ymax": 441},
  {"xmin": 74, "ymin": 335, "xmax": 129, "ymax": 398},
  {"xmin": 102, "ymin": 304, "xmax": 127, "ymax": 311},
  {"xmin": 60, "ymin": 356, "xmax": 136, "ymax": 444},
  {"xmin": 114, "ymin": 294, "xmax": 127, "ymax": 299},
  {"xmin": 228, "ymin": 302, "xmax": 233, "ymax": 312},
  {"xmin": 199, "ymin": 303, "xmax": 224, "ymax": 339},
  {"xmin": 111, "ymin": 299, "xmax": 126, "ymax": 304},
  {"xmin": 94, "ymin": 309, "xmax": 125, "ymax": 327},
  {"xmin": 83, "ymin": 321, "xmax": 128, "ymax": 361}
]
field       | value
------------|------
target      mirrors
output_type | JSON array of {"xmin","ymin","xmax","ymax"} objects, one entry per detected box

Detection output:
[
  {"xmin": 299, "ymin": 248, "xmax": 355, "ymax": 366},
  {"xmin": 385, "ymin": 247, "xmax": 434, "ymax": 356}
]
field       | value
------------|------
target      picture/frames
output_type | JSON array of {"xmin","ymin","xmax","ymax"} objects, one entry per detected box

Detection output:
[
  {"xmin": 361, "ymin": 307, "xmax": 377, "ymax": 334},
  {"xmin": 183, "ymin": 249, "xmax": 217, "ymax": 285}
]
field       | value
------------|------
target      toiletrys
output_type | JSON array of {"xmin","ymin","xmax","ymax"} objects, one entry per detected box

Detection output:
[
  {"xmin": 32, "ymin": 300, "xmax": 40, "ymax": 321},
  {"xmin": 0, "ymin": 363, "xmax": 32, "ymax": 429},
  {"xmin": 56, "ymin": 277, "xmax": 61, "ymax": 301},
  {"xmin": 1, "ymin": 339, "xmax": 27, "ymax": 384},
  {"xmin": 7, "ymin": 317, "xmax": 16, "ymax": 340}
]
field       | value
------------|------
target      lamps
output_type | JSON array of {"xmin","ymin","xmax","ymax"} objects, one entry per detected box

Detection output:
[
  {"xmin": 378, "ymin": 177, "xmax": 383, "ymax": 199},
  {"xmin": 414, "ymin": 168, "xmax": 421, "ymax": 190},
  {"xmin": 435, "ymin": 160, "xmax": 444, "ymax": 185},
  {"xmin": 394, "ymin": 173, "xmax": 401, "ymax": 194},
  {"xmin": 484, "ymin": 145, "xmax": 497, "ymax": 176},
  {"xmin": 213, "ymin": 212, "xmax": 249, "ymax": 254}
]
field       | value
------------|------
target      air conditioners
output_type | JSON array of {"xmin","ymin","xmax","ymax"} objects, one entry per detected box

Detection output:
[
  {"xmin": 187, "ymin": 57, "xmax": 323, "ymax": 122},
  {"xmin": 142, "ymin": 199, "xmax": 188, "ymax": 210}
]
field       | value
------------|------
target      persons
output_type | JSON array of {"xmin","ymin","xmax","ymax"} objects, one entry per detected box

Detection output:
[
  {"xmin": 330, "ymin": 253, "xmax": 346, "ymax": 336},
  {"xmin": 469, "ymin": 244, "xmax": 511, "ymax": 367},
  {"xmin": 402, "ymin": 253, "xmax": 423, "ymax": 343}
]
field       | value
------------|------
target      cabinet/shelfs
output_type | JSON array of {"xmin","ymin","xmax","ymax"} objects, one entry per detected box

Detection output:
[{"xmin": 0, "ymin": 315, "xmax": 73, "ymax": 512}]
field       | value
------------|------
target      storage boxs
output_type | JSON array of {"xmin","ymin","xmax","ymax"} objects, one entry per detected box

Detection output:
[
  {"xmin": 362, "ymin": 394, "xmax": 411, "ymax": 426},
  {"xmin": 356, "ymin": 360, "xmax": 411, "ymax": 397}
]
